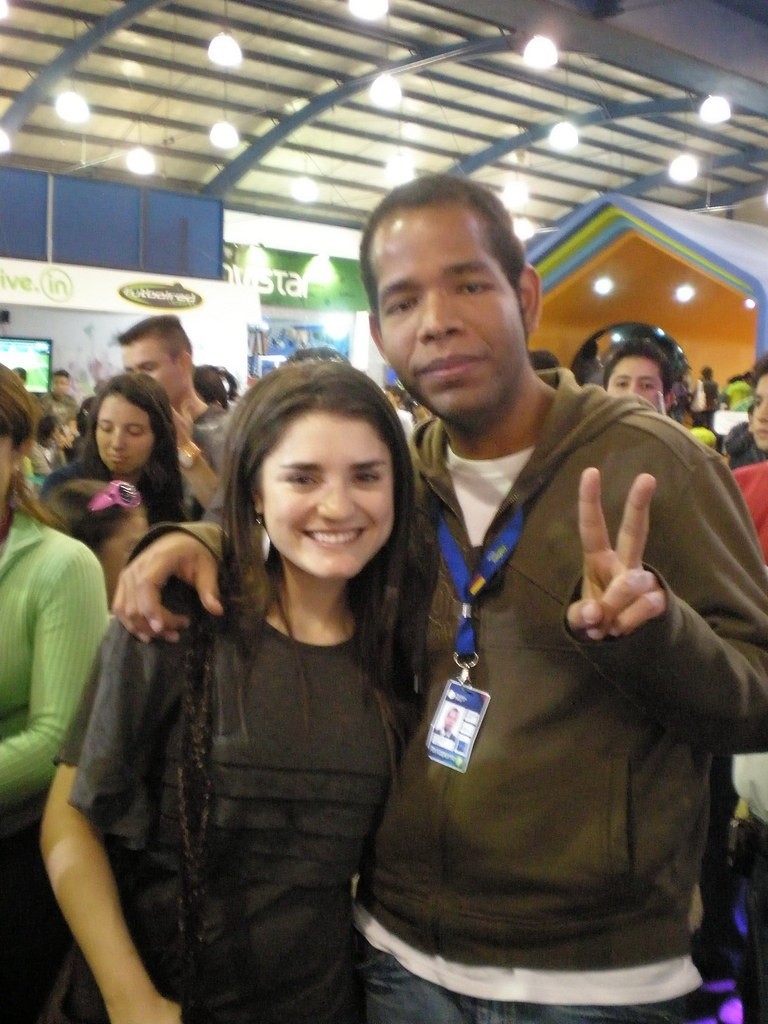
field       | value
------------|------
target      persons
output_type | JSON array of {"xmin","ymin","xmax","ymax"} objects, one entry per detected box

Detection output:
[
  {"xmin": 0, "ymin": 175, "xmax": 768, "ymax": 1024},
  {"xmin": 433, "ymin": 708, "xmax": 459, "ymax": 742}
]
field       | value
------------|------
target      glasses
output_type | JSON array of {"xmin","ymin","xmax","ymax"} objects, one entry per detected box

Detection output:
[{"xmin": 73, "ymin": 478, "xmax": 142, "ymax": 541}]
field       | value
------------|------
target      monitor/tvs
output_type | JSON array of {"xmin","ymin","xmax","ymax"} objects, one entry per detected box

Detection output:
[{"xmin": 0, "ymin": 337, "xmax": 52, "ymax": 394}]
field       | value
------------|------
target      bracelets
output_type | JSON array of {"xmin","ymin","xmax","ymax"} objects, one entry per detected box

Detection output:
[{"xmin": 177, "ymin": 438, "xmax": 201, "ymax": 469}]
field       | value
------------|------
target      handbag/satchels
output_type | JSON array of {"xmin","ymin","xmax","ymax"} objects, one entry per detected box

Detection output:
[
  {"xmin": 38, "ymin": 948, "xmax": 187, "ymax": 1024},
  {"xmin": 684, "ymin": 811, "xmax": 768, "ymax": 1024}
]
